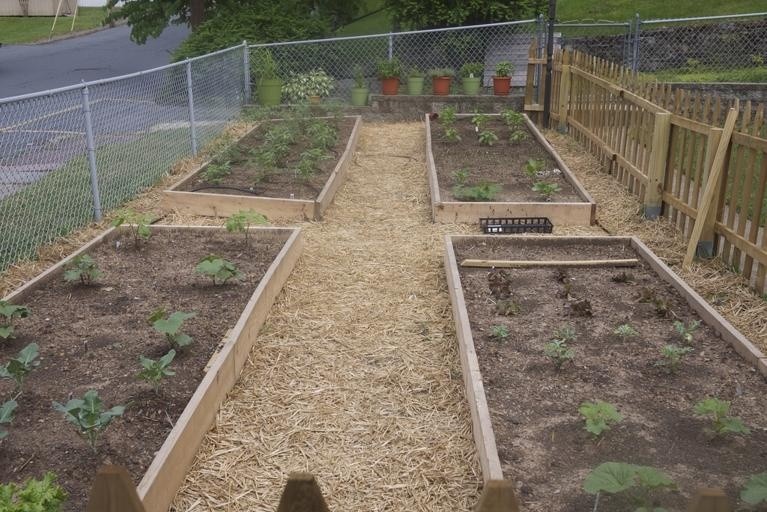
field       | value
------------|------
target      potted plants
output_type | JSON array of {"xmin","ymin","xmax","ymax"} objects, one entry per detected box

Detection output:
[
  {"xmin": 350, "ymin": 53, "xmax": 514, "ymax": 107},
  {"xmin": 249, "ymin": 48, "xmax": 333, "ymax": 106}
]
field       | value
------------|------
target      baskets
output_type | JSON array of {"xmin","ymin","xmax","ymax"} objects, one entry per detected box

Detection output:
[{"xmin": 479, "ymin": 217, "xmax": 553, "ymax": 234}]
